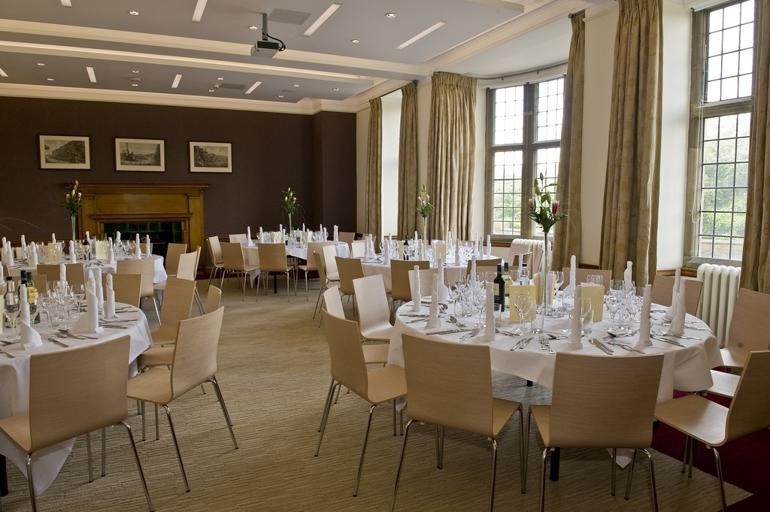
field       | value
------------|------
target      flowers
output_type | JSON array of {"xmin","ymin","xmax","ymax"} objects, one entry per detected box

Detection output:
[
  {"xmin": 527, "ymin": 172, "xmax": 567, "ymax": 315},
  {"xmin": 280, "ymin": 187, "xmax": 299, "ymax": 237},
  {"xmin": 66, "ymin": 180, "xmax": 84, "ymax": 241},
  {"xmin": 416, "ymin": 184, "xmax": 435, "ymax": 257}
]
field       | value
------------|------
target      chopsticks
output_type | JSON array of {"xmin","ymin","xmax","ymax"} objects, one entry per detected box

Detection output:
[
  {"xmin": 420, "ymin": 300, "xmax": 443, "ymax": 305},
  {"xmin": 399, "ymin": 314, "xmax": 441, "ymax": 318}
]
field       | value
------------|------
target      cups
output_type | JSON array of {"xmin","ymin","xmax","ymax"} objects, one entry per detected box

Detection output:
[
  {"xmin": 256, "ymin": 232, "xmax": 261, "ymax": 243},
  {"xmin": 39, "ymin": 290, "xmax": 56, "ymax": 334},
  {"xmin": 453, "ymin": 300, "xmax": 470, "ymax": 337},
  {"xmin": 562, "ymin": 292, "xmax": 578, "ymax": 320},
  {"xmin": 579, "ymin": 296, "xmax": 592, "ymax": 338},
  {"xmin": 402, "ymin": 245, "xmax": 408, "ymax": 260},
  {"xmin": 58, "ymin": 240, "xmax": 65, "ymax": 252},
  {"xmin": 624, "ymin": 296, "xmax": 632, "ymax": 321},
  {"xmin": 314, "ymin": 230, "xmax": 320, "ymax": 242},
  {"xmin": 494, "ymin": 303, "xmax": 503, "ymax": 330},
  {"xmin": 379, "ymin": 241, "xmax": 385, "ymax": 257},
  {"xmin": 605, "ymin": 290, "xmax": 623, "ymax": 332},
  {"xmin": 70, "ymin": 283, "xmax": 86, "ymax": 319},
  {"xmin": 287, "ymin": 233, "xmax": 296, "ymax": 248},
  {"xmin": 122, "ymin": 239, "xmax": 130, "ymax": 261},
  {"xmin": 52, "ymin": 278, "xmax": 68, "ymax": 301},
  {"xmin": 3, "ymin": 295, "xmax": 23, "ymax": 344},
  {"xmin": 36, "ymin": 240, "xmax": 45, "ymax": 251},
  {"xmin": 513, "ymin": 292, "xmax": 533, "ymax": 338},
  {"xmin": 531, "ymin": 314, "xmax": 544, "ymax": 334},
  {"xmin": 45, "ymin": 280, "xmax": 57, "ymax": 311},
  {"xmin": 322, "ymin": 232, "xmax": 328, "ymax": 242},
  {"xmin": 626, "ymin": 295, "xmax": 640, "ymax": 321},
  {"xmin": 81, "ymin": 245, "xmax": 90, "ymax": 263},
  {"xmin": 555, "ymin": 270, "xmax": 566, "ymax": 303}
]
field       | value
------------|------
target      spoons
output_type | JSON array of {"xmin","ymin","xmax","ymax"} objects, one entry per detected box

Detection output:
[
  {"xmin": 494, "ymin": 328, "xmax": 512, "ymax": 337},
  {"xmin": 607, "ymin": 332, "xmax": 637, "ymax": 338},
  {"xmin": 448, "ymin": 315, "xmax": 465, "ymax": 331},
  {"xmin": 547, "ymin": 333, "xmax": 584, "ymax": 339}
]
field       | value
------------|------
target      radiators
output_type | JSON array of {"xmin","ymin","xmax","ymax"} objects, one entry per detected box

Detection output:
[
  {"xmin": 509, "ymin": 238, "xmax": 546, "ymax": 277},
  {"xmin": 694, "ymin": 263, "xmax": 742, "ymax": 353}
]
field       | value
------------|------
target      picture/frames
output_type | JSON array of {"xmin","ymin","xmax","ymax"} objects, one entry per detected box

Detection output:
[
  {"xmin": 36, "ymin": 132, "xmax": 91, "ymax": 173},
  {"xmin": 188, "ymin": 141, "xmax": 233, "ymax": 174},
  {"xmin": 114, "ymin": 137, "xmax": 165, "ymax": 172}
]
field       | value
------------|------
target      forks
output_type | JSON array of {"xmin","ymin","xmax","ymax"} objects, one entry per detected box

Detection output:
[
  {"xmin": 538, "ymin": 336, "xmax": 546, "ymax": 350},
  {"xmin": 543, "ymin": 337, "xmax": 555, "ymax": 354},
  {"xmin": 99, "ymin": 318, "xmax": 138, "ymax": 323},
  {"xmin": 652, "ymin": 329, "xmax": 693, "ymax": 339},
  {"xmin": 61, "ymin": 330, "xmax": 85, "ymax": 340}
]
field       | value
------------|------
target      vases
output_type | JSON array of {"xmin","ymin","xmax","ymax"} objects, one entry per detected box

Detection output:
[
  {"xmin": 542, "ymin": 233, "xmax": 551, "ymax": 315},
  {"xmin": 421, "ymin": 217, "xmax": 428, "ymax": 258},
  {"xmin": 288, "ymin": 213, "xmax": 292, "ymax": 237},
  {"xmin": 71, "ymin": 216, "xmax": 76, "ymax": 240}
]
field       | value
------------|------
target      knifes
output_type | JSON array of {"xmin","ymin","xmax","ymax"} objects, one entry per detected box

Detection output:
[
  {"xmin": 406, "ymin": 319, "xmax": 424, "ymax": 324},
  {"xmin": 47, "ymin": 337, "xmax": 69, "ymax": 347},
  {"xmin": 650, "ymin": 334, "xmax": 687, "ymax": 348},
  {"xmin": 520, "ymin": 337, "xmax": 534, "ymax": 349},
  {"xmin": 588, "ymin": 339, "xmax": 612, "ymax": 355},
  {"xmin": 76, "ymin": 335, "xmax": 98, "ymax": 340},
  {"xmin": 593, "ymin": 338, "xmax": 614, "ymax": 353},
  {"xmin": 440, "ymin": 329, "xmax": 472, "ymax": 335},
  {"xmin": 511, "ymin": 338, "xmax": 527, "ymax": 350},
  {"xmin": 98, "ymin": 324, "xmax": 128, "ymax": 329},
  {"xmin": 426, "ymin": 330, "xmax": 453, "ymax": 335}
]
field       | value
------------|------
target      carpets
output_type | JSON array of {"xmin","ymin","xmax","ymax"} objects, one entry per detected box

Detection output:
[{"xmin": 651, "ymin": 369, "xmax": 768, "ymax": 511}]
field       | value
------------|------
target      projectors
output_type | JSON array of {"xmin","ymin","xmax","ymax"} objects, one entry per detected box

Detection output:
[{"xmin": 250, "ymin": 35, "xmax": 286, "ymax": 59}]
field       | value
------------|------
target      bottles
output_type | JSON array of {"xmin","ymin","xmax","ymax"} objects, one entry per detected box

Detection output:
[
  {"xmin": 82, "ymin": 234, "xmax": 90, "ymax": 261},
  {"xmin": 402, "ymin": 234, "xmax": 409, "ymax": 260},
  {"xmin": 518, "ymin": 263, "xmax": 530, "ymax": 285},
  {"xmin": 18, "ymin": 270, "xmax": 27, "ymax": 302},
  {"xmin": 494, "ymin": 264, "xmax": 504, "ymax": 312},
  {"xmin": 4, "ymin": 277, "xmax": 19, "ymax": 312},
  {"xmin": 285, "ymin": 227, "xmax": 288, "ymax": 246},
  {"xmin": 501, "ymin": 262, "xmax": 514, "ymax": 310},
  {"xmin": 24, "ymin": 272, "xmax": 39, "ymax": 322}
]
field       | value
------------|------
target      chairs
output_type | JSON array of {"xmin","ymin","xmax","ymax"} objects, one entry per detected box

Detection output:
[
  {"xmin": 313, "ymin": 250, "xmax": 340, "ymax": 328},
  {"xmin": 36, "ymin": 264, "xmax": 87, "ymax": 299},
  {"xmin": 527, "ymin": 353, "xmax": 664, "ymax": 511},
  {"xmin": 338, "ymin": 231, "xmax": 355, "ymax": 247},
  {"xmin": 295, "ymin": 242, "xmax": 336, "ymax": 301},
  {"xmin": 134, "ymin": 243, "xmax": 153, "ymax": 252},
  {"xmin": 208, "ymin": 235, "xmax": 241, "ymax": 294},
  {"xmin": 0, "ymin": 334, "xmax": 153, "ymax": 511},
  {"xmin": 649, "ymin": 273, "xmax": 701, "ymax": 319},
  {"xmin": 511, "ymin": 253, "xmax": 532, "ymax": 270},
  {"xmin": 101, "ymin": 305, "xmax": 240, "ymax": 494},
  {"xmin": 220, "ymin": 243, "xmax": 259, "ymax": 301},
  {"xmin": 145, "ymin": 276, "xmax": 207, "ymax": 399},
  {"xmin": 116, "ymin": 258, "xmax": 166, "ymax": 348},
  {"xmin": 152, "ymin": 250, "xmax": 202, "ymax": 319},
  {"xmin": 351, "ymin": 242, "xmax": 366, "ymax": 257},
  {"xmin": 465, "ymin": 259, "xmax": 503, "ymax": 282},
  {"xmin": 205, "ymin": 238, "xmax": 214, "ymax": 292},
  {"xmin": 709, "ymin": 286, "xmax": 769, "ymax": 368},
  {"xmin": 102, "ymin": 272, "xmax": 142, "ymax": 304},
  {"xmin": 229, "ymin": 233, "xmax": 249, "ymax": 246},
  {"xmin": 352, "ymin": 274, "xmax": 396, "ymax": 344},
  {"xmin": 163, "ymin": 242, "xmax": 188, "ymax": 277},
  {"xmin": 624, "ymin": 351, "xmax": 769, "ymax": 511},
  {"xmin": 11, "ymin": 275, "xmax": 47, "ymax": 296},
  {"xmin": 335, "ymin": 255, "xmax": 364, "ymax": 317},
  {"xmin": 560, "ymin": 268, "xmax": 616, "ymax": 295},
  {"xmin": 194, "ymin": 244, "xmax": 207, "ymax": 315},
  {"xmin": 431, "ymin": 239, "xmax": 445, "ymax": 248},
  {"xmin": 390, "ymin": 333, "xmax": 527, "ymax": 512},
  {"xmin": 398, "ymin": 240, "xmax": 405, "ymax": 254},
  {"xmin": 313, "ymin": 307, "xmax": 440, "ymax": 497},
  {"xmin": 475, "ymin": 267, "xmax": 519, "ymax": 286},
  {"xmin": 2, "ymin": 263, "xmax": 22, "ymax": 279},
  {"xmin": 256, "ymin": 243, "xmax": 294, "ymax": 304},
  {"xmin": 390, "ymin": 259, "xmax": 430, "ymax": 313},
  {"xmin": 135, "ymin": 284, "xmax": 221, "ymax": 440},
  {"xmin": 317, "ymin": 283, "xmax": 404, "ymax": 435},
  {"xmin": 682, "ymin": 369, "xmax": 742, "ymax": 473},
  {"xmin": 408, "ymin": 267, "xmax": 444, "ymax": 296},
  {"xmin": 323, "ymin": 244, "xmax": 340, "ymax": 286}
]
